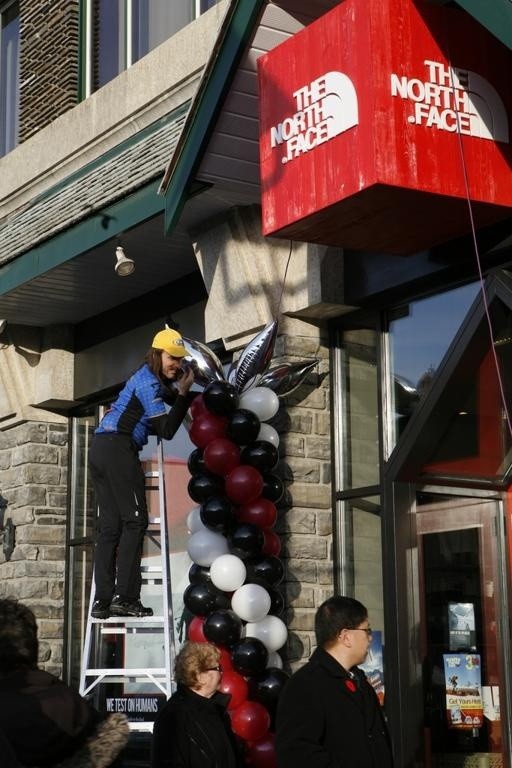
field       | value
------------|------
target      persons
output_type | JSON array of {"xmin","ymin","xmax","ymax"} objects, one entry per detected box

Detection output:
[
  {"xmin": 151, "ymin": 641, "xmax": 239, "ymax": 768},
  {"xmin": 88, "ymin": 323, "xmax": 194, "ymax": 621},
  {"xmin": 275, "ymin": 596, "xmax": 394, "ymax": 768},
  {"xmin": 0, "ymin": 597, "xmax": 130, "ymax": 768}
]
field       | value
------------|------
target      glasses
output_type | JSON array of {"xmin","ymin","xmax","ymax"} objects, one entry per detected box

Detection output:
[
  {"xmin": 348, "ymin": 627, "xmax": 372, "ymax": 635},
  {"xmin": 199, "ymin": 665, "xmax": 223, "ymax": 673}
]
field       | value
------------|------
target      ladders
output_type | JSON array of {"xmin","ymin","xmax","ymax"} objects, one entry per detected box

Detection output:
[{"xmin": 79, "ymin": 402, "xmax": 173, "ymax": 704}]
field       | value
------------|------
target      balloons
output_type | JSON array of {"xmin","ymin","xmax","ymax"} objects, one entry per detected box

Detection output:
[{"xmin": 176, "ymin": 315, "xmax": 321, "ymax": 767}]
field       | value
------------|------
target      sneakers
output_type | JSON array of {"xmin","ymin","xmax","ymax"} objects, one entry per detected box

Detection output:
[{"xmin": 91, "ymin": 593, "xmax": 153, "ymax": 619}]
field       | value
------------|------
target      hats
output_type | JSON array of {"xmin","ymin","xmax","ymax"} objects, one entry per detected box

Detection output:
[{"xmin": 151, "ymin": 328, "xmax": 194, "ymax": 359}]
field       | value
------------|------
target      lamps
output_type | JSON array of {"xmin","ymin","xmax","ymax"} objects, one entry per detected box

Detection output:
[{"xmin": 112, "ymin": 244, "xmax": 136, "ymax": 277}]
